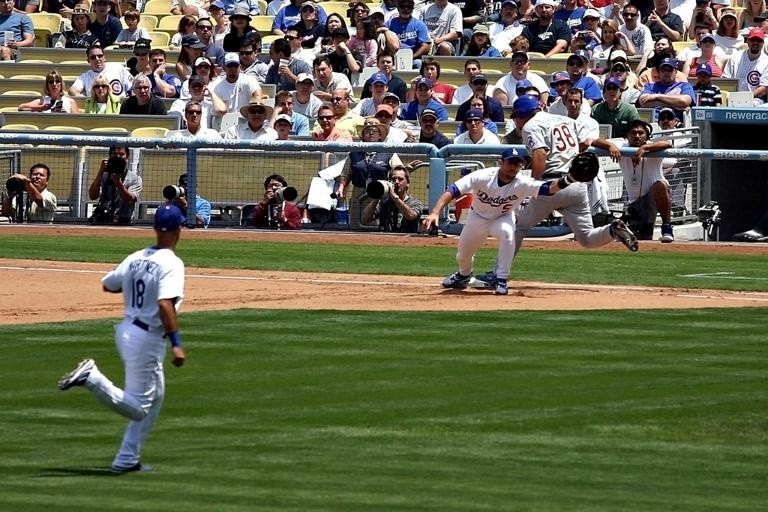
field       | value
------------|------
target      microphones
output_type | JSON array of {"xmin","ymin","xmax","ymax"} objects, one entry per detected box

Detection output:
[{"xmin": 330, "ymin": 193, "xmax": 346, "ymax": 199}]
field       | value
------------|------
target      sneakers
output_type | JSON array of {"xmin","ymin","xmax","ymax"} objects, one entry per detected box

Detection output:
[
  {"xmin": 443, "ymin": 272, "xmax": 509, "ymax": 295},
  {"xmin": 660, "ymin": 226, "xmax": 674, "ymax": 242},
  {"xmin": 611, "ymin": 219, "xmax": 638, "ymax": 252},
  {"xmin": 57, "ymin": 358, "xmax": 96, "ymax": 390},
  {"xmin": 111, "ymin": 461, "xmax": 141, "ymax": 473}
]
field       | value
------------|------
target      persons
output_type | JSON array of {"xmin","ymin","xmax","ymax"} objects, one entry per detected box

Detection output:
[
  {"xmin": 476, "ymin": 95, "xmax": 639, "ymax": 281},
  {"xmin": 0, "ymin": 0, "xmax": 768, "ymax": 243},
  {"xmin": 58, "ymin": 204, "xmax": 186, "ymax": 473},
  {"xmin": 420, "ymin": 148, "xmax": 598, "ymax": 294}
]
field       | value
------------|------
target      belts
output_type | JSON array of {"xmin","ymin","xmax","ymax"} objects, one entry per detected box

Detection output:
[{"xmin": 130, "ymin": 317, "xmax": 168, "ymax": 338}]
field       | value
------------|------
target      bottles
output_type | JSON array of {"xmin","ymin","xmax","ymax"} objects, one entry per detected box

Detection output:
[{"xmin": 43, "ymin": 96, "xmax": 51, "ymax": 113}]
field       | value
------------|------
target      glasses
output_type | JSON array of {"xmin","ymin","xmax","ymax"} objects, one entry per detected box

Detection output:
[
  {"xmin": 95, "ymin": 84, "xmax": 108, "ymax": 89},
  {"xmin": 319, "ymin": 97, "xmax": 342, "ymax": 121},
  {"xmin": 196, "ymin": 24, "xmax": 212, "ymax": 30},
  {"xmin": 250, "ymin": 109, "xmax": 265, "ymax": 115},
  {"xmin": 47, "ymin": 80, "xmax": 62, "ymax": 84},
  {"xmin": 240, "ymin": 50, "xmax": 253, "ymax": 56},
  {"xmin": 90, "ymin": 55, "xmax": 103, "ymax": 60},
  {"xmin": 188, "ymin": 110, "xmax": 203, "ymax": 117},
  {"xmin": 135, "ymin": 86, "xmax": 149, "ymax": 92},
  {"xmin": 283, "ymin": 35, "xmax": 300, "ymax": 41}
]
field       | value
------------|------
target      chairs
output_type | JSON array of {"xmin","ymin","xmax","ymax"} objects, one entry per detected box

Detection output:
[{"xmin": 0, "ymin": 0, "xmax": 768, "ymax": 146}]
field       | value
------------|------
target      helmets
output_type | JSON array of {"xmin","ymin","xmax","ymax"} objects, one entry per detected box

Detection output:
[{"xmin": 155, "ymin": 204, "xmax": 187, "ymax": 230}]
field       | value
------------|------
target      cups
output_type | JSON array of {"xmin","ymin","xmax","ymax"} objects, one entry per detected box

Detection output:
[
  {"xmin": 278, "ymin": 58, "xmax": 289, "ymax": 75},
  {"xmin": 4, "ymin": 30, "xmax": 14, "ymax": 48}
]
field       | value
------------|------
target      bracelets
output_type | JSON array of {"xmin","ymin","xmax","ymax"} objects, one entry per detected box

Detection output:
[{"xmin": 168, "ymin": 331, "xmax": 182, "ymax": 347}]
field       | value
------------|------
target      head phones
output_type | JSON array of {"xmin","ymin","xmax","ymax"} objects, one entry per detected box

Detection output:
[{"xmin": 622, "ymin": 121, "xmax": 653, "ymax": 140}]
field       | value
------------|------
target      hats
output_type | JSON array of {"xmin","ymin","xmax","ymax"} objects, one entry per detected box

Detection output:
[
  {"xmin": 502, "ymin": 148, "xmax": 525, "ymax": 164},
  {"xmin": 274, "ymin": 113, "xmax": 291, "ymax": 126},
  {"xmin": 182, "ymin": 35, "xmax": 241, "ymax": 87},
  {"xmin": 300, "ymin": 2, "xmax": 319, "ymax": 11},
  {"xmin": 228, "ymin": 7, "xmax": 253, "ymax": 22},
  {"xmin": 295, "ymin": 72, "xmax": 314, "ymax": 85},
  {"xmin": 356, "ymin": 73, "xmax": 436, "ymax": 138},
  {"xmin": 63, "ymin": 4, "xmax": 97, "ymax": 25},
  {"xmin": 209, "ymin": 2, "xmax": 224, "ymax": 11},
  {"xmin": 467, "ymin": 0, "xmax": 768, "ymax": 120},
  {"xmin": 134, "ymin": 38, "xmax": 150, "ymax": 55},
  {"xmin": 239, "ymin": 97, "xmax": 273, "ymax": 122}
]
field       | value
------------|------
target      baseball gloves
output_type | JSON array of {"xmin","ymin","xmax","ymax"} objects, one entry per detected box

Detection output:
[{"xmin": 571, "ymin": 152, "xmax": 599, "ymax": 183}]
